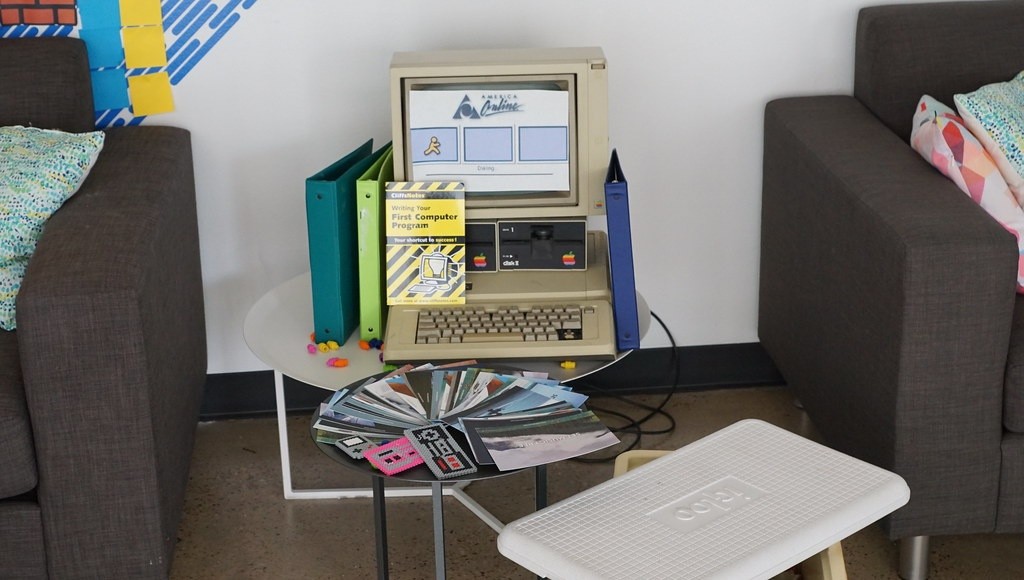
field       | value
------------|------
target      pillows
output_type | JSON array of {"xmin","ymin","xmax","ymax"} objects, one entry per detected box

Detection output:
[
  {"xmin": 910, "ymin": 95, "xmax": 1024, "ymax": 292},
  {"xmin": 0, "ymin": 125, "xmax": 105, "ymax": 331},
  {"xmin": 953, "ymin": 71, "xmax": 1024, "ymax": 207}
]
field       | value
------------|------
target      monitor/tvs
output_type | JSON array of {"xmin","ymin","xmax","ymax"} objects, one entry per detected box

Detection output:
[{"xmin": 390, "ymin": 45, "xmax": 610, "ymax": 220}]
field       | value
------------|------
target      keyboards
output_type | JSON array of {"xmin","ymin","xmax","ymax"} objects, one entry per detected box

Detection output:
[{"xmin": 383, "ymin": 299, "xmax": 616, "ymax": 365}]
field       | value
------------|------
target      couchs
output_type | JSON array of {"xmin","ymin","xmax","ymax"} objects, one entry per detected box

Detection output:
[
  {"xmin": 758, "ymin": 0, "xmax": 1024, "ymax": 580},
  {"xmin": 0, "ymin": 36, "xmax": 208, "ymax": 580}
]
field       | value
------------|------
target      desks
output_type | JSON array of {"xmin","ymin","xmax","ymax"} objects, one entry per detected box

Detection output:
[
  {"xmin": 309, "ymin": 359, "xmax": 620, "ymax": 580},
  {"xmin": 241, "ymin": 269, "xmax": 651, "ymax": 536}
]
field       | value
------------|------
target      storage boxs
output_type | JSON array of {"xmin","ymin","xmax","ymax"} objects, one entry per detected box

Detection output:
[{"xmin": 497, "ymin": 418, "xmax": 910, "ymax": 580}]
